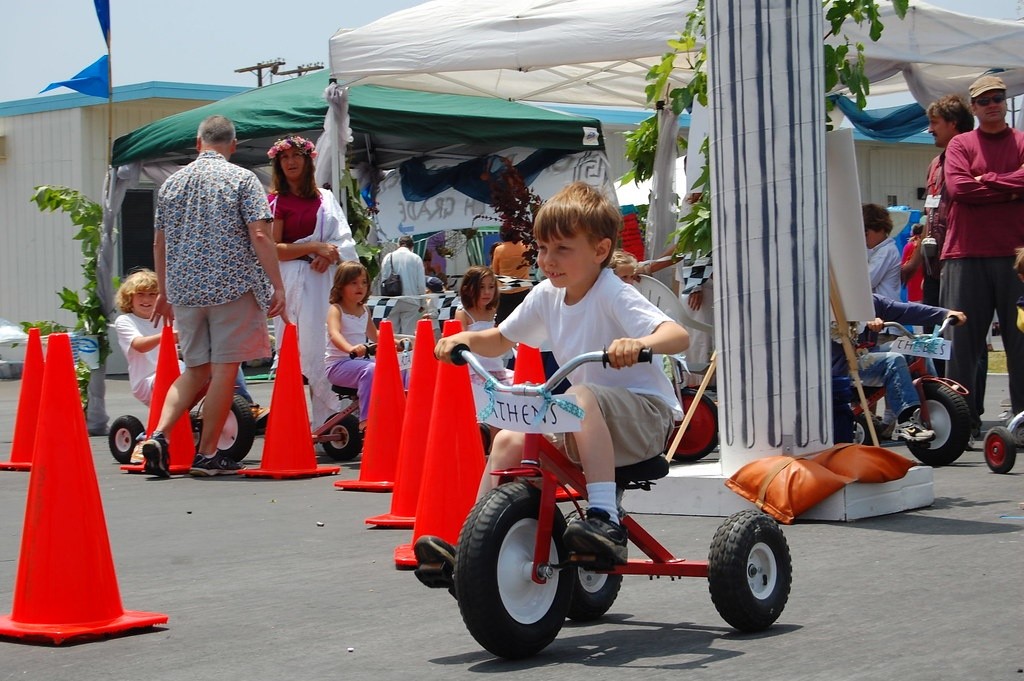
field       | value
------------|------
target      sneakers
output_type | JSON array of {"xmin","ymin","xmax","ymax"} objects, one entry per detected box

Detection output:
[
  {"xmin": 190, "ymin": 447, "xmax": 247, "ymax": 477},
  {"xmin": 415, "ymin": 536, "xmax": 459, "ymax": 604},
  {"xmin": 144, "ymin": 430, "xmax": 170, "ymax": 479},
  {"xmin": 890, "ymin": 408, "xmax": 937, "ymax": 444},
  {"xmin": 563, "ymin": 507, "xmax": 630, "ymax": 568}
]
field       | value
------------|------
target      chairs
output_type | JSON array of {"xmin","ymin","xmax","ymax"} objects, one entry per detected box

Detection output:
[{"xmin": 633, "ymin": 273, "xmax": 715, "ymax": 375}]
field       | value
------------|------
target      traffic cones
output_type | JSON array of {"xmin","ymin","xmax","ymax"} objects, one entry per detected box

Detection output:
[
  {"xmin": 0, "ymin": 332, "xmax": 169, "ymax": 646},
  {"xmin": 512, "ymin": 341, "xmax": 585, "ymax": 501},
  {"xmin": 236, "ymin": 322, "xmax": 342, "ymax": 480},
  {"xmin": 1, "ymin": 327, "xmax": 47, "ymax": 470},
  {"xmin": 121, "ymin": 326, "xmax": 196, "ymax": 474},
  {"xmin": 334, "ymin": 322, "xmax": 407, "ymax": 489},
  {"xmin": 363, "ymin": 319, "xmax": 440, "ymax": 528},
  {"xmin": 394, "ymin": 320, "xmax": 488, "ymax": 567}
]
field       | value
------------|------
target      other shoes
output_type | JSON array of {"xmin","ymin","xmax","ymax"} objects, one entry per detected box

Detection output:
[{"xmin": 251, "ymin": 404, "xmax": 270, "ymax": 425}]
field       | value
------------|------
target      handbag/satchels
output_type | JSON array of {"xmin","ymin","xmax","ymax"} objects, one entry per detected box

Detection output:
[{"xmin": 380, "ymin": 272, "xmax": 402, "ymax": 296}]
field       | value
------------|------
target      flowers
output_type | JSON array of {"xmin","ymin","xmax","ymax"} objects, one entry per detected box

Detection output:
[{"xmin": 266, "ymin": 135, "xmax": 318, "ymax": 160}]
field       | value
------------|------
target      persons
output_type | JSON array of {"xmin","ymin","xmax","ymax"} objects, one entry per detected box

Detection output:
[
  {"xmin": 833, "ymin": 75, "xmax": 1024, "ymax": 443},
  {"xmin": 115, "ymin": 115, "xmax": 360, "ymax": 479},
  {"xmin": 325, "ymin": 225, "xmax": 531, "ymax": 453},
  {"xmin": 606, "ymin": 156, "xmax": 717, "ymax": 391},
  {"xmin": 414, "ymin": 182, "xmax": 690, "ymax": 600}
]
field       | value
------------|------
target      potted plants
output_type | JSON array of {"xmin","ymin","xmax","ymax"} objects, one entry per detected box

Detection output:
[{"xmin": 18, "ymin": 183, "xmax": 124, "ymax": 421}]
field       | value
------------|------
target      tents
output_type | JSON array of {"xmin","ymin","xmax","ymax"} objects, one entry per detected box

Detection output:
[
  {"xmin": 327, "ymin": 0, "xmax": 1024, "ymax": 203},
  {"xmin": 88, "ymin": 66, "xmax": 621, "ymax": 447}
]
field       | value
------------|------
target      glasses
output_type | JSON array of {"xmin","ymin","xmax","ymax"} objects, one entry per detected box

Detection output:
[{"xmin": 975, "ymin": 94, "xmax": 1005, "ymax": 107}]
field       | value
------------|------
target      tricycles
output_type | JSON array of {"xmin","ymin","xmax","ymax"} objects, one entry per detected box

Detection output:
[
  {"xmin": 983, "ymin": 411, "xmax": 1024, "ymax": 474},
  {"xmin": 107, "ymin": 380, "xmax": 256, "ymax": 465},
  {"xmin": 414, "ymin": 341, "xmax": 792, "ymax": 662},
  {"xmin": 660, "ymin": 387, "xmax": 721, "ymax": 463},
  {"xmin": 849, "ymin": 314, "xmax": 972, "ymax": 464},
  {"xmin": 309, "ymin": 338, "xmax": 411, "ymax": 463}
]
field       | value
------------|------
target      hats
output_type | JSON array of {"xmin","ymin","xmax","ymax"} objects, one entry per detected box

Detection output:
[{"xmin": 968, "ymin": 76, "xmax": 1007, "ymax": 98}]
